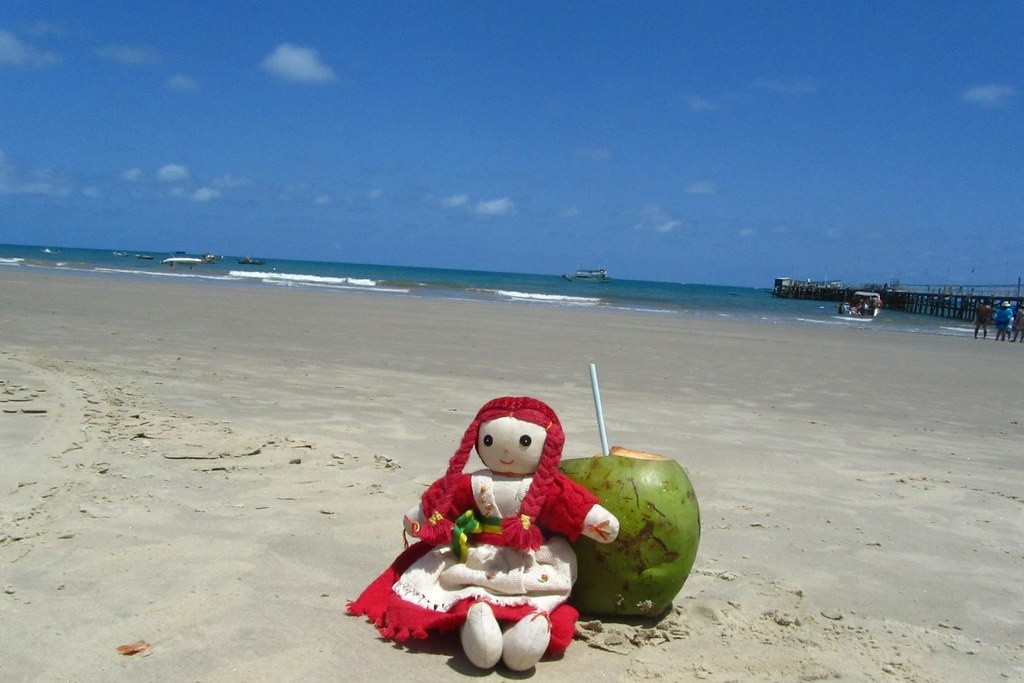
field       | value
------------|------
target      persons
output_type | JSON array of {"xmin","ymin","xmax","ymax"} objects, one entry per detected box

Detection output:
[
  {"xmin": 994, "ymin": 301, "xmax": 1024, "ymax": 343},
  {"xmin": 346, "ymin": 397, "xmax": 621, "ymax": 672},
  {"xmin": 974, "ymin": 299, "xmax": 992, "ymax": 340}
]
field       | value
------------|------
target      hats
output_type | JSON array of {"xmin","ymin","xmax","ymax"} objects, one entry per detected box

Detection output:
[{"xmin": 1001, "ymin": 301, "xmax": 1010, "ymax": 307}]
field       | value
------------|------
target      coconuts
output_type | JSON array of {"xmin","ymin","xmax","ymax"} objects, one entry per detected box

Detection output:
[{"xmin": 553, "ymin": 445, "xmax": 701, "ymax": 618}]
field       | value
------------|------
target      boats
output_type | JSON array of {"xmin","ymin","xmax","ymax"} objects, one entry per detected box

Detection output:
[
  {"xmin": 839, "ymin": 291, "xmax": 880, "ymax": 316},
  {"xmin": 42, "ymin": 250, "xmax": 62, "ymax": 254},
  {"xmin": 201, "ymin": 252, "xmax": 224, "ymax": 264},
  {"xmin": 138, "ymin": 255, "xmax": 153, "ymax": 259},
  {"xmin": 237, "ymin": 257, "xmax": 265, "ymax": 265},
  {"xmin": 562, "ymin": 270, "xmax": 611, "ymax": 283},
  {"xmin": 160, "ymin": 257, "xmax": 203, "ymax": 265},
  {"xmin": 112, "ymin": 252, "xmax": 128, "ymax": 257}
]
